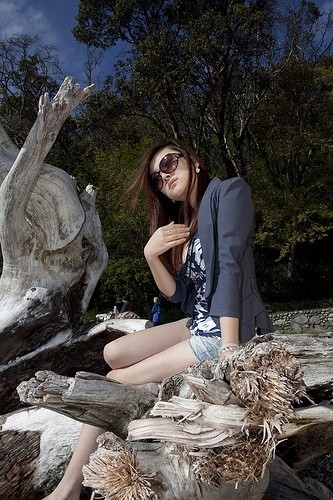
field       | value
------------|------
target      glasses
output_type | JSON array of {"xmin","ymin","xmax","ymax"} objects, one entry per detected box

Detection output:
[{"xmin": 149, "ymin": 153, "xmax": 184, "ymax": 192}]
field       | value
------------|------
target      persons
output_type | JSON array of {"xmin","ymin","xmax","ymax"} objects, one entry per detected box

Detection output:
[
  {"xmin": 120, "ymin": 296, "xmax": 134, "ymax": 312},
  {"xmin": 41, "ymin": 139, "xmax": 276, "ymax": 500},
  {"xmin": 151, "ymin": 297, "xmax": 162, "ymax": 327}
]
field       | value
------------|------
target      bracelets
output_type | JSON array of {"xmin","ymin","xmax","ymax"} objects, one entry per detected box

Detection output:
[{"xmin": 218, "ymin": 343, "xmax": 242, "ymax": 355}]
